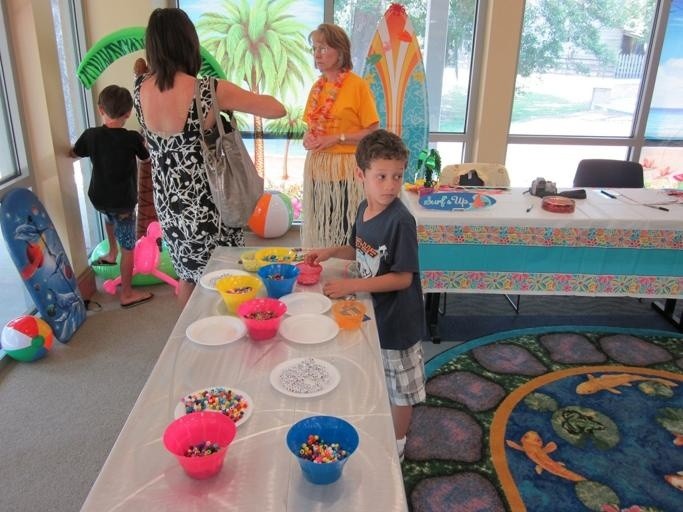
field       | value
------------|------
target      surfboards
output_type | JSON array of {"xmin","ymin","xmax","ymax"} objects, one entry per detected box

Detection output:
[
  {"xmin": 419, "ymin": 192, "xmax": 497, "ymax": 212},
  {"xmin": 361, "ymin": 5, "xmax": 429, "ymax": 184}
]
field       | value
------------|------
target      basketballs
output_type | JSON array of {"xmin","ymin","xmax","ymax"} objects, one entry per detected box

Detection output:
[
  {"xmin": 2, "ymin": 314, "xmax": 54, "ymax": 363},
  {"xmin": 249, "ymin": 191, "xmax": 293, "ymax": 238}
]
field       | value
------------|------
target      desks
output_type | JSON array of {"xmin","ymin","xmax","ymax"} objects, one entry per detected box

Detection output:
[
  {"xmin": 401, "ymin": 186, "xmax": 683, "ymax": 344},
  {"xmin": 82, "ymin": 246, "xmax": 407, "ymax": 512}
]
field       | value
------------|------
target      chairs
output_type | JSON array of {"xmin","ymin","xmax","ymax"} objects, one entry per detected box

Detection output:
[
  {"xmin": 439, "ymin": 163, "xmax": 521, "ymax": 316},
  {"xmin": 574, "ymin": 159, "xmax": 645, "ymax": 187}
]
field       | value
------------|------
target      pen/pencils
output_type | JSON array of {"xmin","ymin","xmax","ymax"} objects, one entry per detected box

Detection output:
[
  {"xmin": 601, "ymin": 190, "xmax": 616, "ymax": 199},
  {"xmin": 644, "ymin": 204, "xmax": 669, "ymax": 211},
  {"xmin": 526, "ymin": 204, "xmax": 534, "ymax": 212}
]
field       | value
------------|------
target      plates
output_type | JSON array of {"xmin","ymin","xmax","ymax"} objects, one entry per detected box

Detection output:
[
  {"xmin": 270, "ymin": 357, "xmax": 341, "ymax": 397},
  {"xmin": 187, "ymin": 315, "xmax": 247, "ymax": 346},
  {"xmin": 175, "ymin": 386, "xmax": 254, "ymax": 428},
  {"xmin": 199, "ymin": 269, "xmax": 250, "ymax": 291}
]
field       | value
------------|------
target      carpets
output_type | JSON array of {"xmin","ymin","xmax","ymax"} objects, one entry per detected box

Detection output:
[{"xmin": 402, "ymin": 325, "xmax": 683, "ymax": 512}]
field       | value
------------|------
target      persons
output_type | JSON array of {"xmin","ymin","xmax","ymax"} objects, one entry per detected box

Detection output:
[
  {"xmin": 69, "ymin": 84, "xmax": 154, "ymax": 309},
  {"xmin": 303, "ymin": 23, "xmax": 381, "ymax": 250},
  {"xmin": 131, "ymin": 8, "xmax": 286, "ymax": 322},
  {"xmin": 305, "ymin": 128, "xmax": 425, "ymax": 462}
]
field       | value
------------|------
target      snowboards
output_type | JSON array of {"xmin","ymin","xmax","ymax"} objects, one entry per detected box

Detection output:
[{"xmin": 1, "ymin": 189, "xmax": 86, "ymax": 343}]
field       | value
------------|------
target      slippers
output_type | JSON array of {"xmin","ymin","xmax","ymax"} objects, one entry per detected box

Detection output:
[
  {"xmin": 91, "ymin": 256, "xmax": 117, "ymax": 267},
  {"xmin": 120, "ymin": 290, "xmax": 154, "ymax": 309}
]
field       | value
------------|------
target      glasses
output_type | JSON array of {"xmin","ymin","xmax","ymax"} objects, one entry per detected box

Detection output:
[{"xmin": 308, "ymin": 45, "xmax": 330, "ymax": 55}]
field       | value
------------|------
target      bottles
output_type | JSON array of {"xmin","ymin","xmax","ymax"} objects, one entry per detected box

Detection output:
[
  {"xmin": 417, "ymin": 147, "xmax": 441, "ymax": 187},
  {"xmin": 532, "ymin": 177, "xmax": 553, "ymax": 196}
]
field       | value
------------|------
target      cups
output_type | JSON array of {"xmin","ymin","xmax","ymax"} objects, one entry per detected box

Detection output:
[
  {"xmin": 217, "ymin": 248, "xmax": 366, "ymax": 343},
  {"xmin": 164, "ymin": 411, "xmax": 359, "ymax": 485}
]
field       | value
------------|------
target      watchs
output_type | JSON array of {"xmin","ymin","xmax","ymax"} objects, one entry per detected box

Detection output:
[{"xmin": 339, "ymin": 133, "xmax": 346, "ymax": 145}]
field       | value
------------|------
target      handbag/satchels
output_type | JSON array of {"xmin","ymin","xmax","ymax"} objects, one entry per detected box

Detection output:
[{"xmin": 194, "ymin": 76, "xmax": 265, "ymax": 229}]
